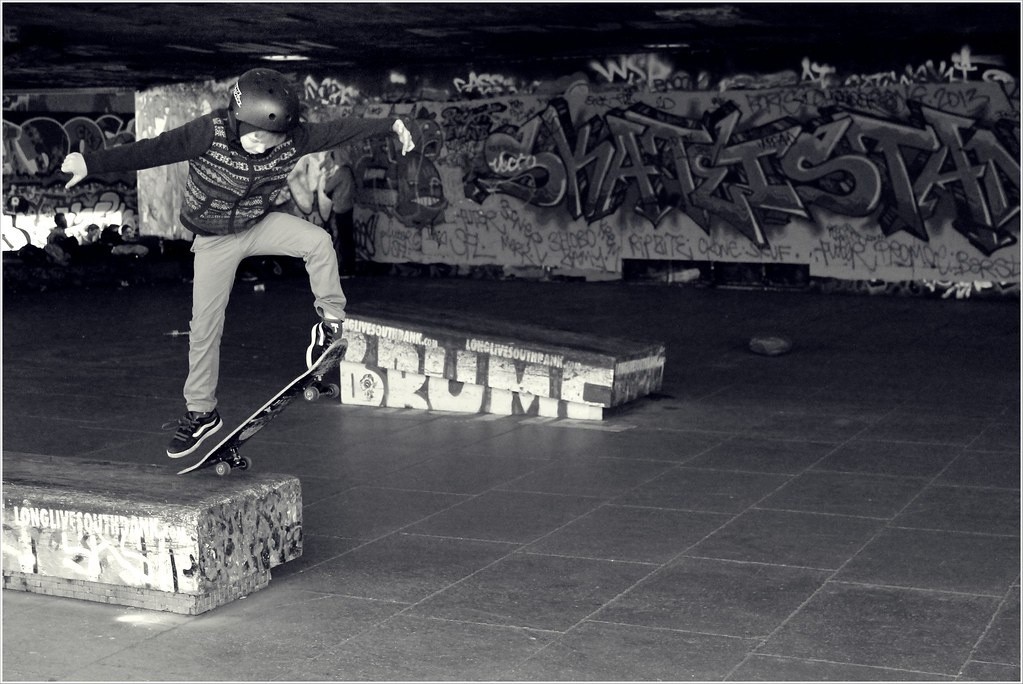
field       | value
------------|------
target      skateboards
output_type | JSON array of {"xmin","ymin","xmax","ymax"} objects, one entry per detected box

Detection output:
[{"xmin": 177, "ymin": 338, "xmax": 349, "ymax": 476}]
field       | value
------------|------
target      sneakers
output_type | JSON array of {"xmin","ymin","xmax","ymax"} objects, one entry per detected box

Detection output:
[
  {"xmin": 162, "ymin": 408, "xmax": 223, "ymax": 457},
  {"xmin": 306, "ymin": 320, "xmax": 339, "ymax": 369}
]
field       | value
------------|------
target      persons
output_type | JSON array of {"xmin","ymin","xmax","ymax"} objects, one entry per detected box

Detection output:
[
  {"xmin": 61, "ymin": 67, "xmax": 416, "ymax": 461},
  {"xmin": 50, "ymin": 212, "xmax": 147, "ymax": 289}
]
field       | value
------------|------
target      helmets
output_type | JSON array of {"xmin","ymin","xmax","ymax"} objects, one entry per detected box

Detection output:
[{"xmin": 229, "ymin": 67, "xmax": 300, "ymax": 132}]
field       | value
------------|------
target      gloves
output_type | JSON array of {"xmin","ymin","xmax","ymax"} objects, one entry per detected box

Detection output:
[
  {"xmin": 60, "ymin": 151, "xmax": 88, "ymax": 189},
  {"xmin": 392, "ymin": 119, "xmax": 416, "ymax": 156}
]
